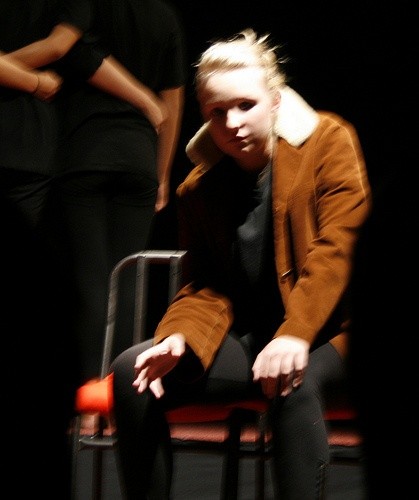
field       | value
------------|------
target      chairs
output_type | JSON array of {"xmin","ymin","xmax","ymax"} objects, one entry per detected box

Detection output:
[{"xmin": 75, "ymin": 248, "xmax": 360, "ymax": 500}]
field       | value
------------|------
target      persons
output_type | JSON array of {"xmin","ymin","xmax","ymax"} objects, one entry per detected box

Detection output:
[
  {"xmin": 0, "ymin": 53, "xmax": 61, "ymax": 100},
  {"xmin": 7, "ymin": 2, "xmax": 187, "ymax": 380},
  {"xmin": 113, "ymin": 31, "xmax": 369, "ymax": 500},
  {"xmin": 0, "ymin": 0, "xmax": 168, "ymax": 491}
]
